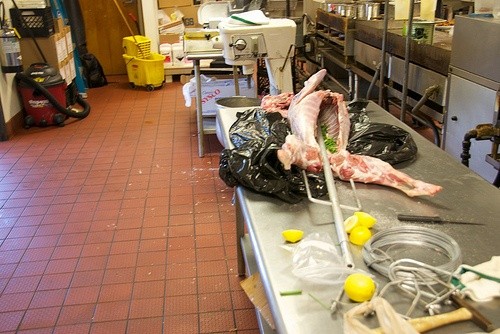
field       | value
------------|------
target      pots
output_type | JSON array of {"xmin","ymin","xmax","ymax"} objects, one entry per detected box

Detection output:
[{"xmin": 326, "ymin": 2, "xmax": 381, "ymax": 21}]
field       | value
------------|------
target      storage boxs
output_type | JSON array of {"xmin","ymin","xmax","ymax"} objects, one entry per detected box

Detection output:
[
  {"xmin": 157, "ymin": 0, "xmax": 197, "ymax": 30},
  {"xmin": 9, "ymin": 8, "xmax": 55, "ymax": 38}
]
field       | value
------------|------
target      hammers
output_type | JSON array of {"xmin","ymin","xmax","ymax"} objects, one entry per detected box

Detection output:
[{"xmin": 371, "ymin": 292, "xmax": 495, "ymax": 333}]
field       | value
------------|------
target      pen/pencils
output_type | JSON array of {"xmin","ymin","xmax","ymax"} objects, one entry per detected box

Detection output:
[{"xmin": 328, "ymin": 283, "xmax": 343, "ymax": 316}]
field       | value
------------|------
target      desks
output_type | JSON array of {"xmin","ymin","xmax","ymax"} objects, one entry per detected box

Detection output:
[
  {"xmin": 215, "ymin": 98, "xmax": 500, "ymax": 334},
  {"xmin": 186, "ymin": 53, "xmax": 255, "ymax": 159}
]
